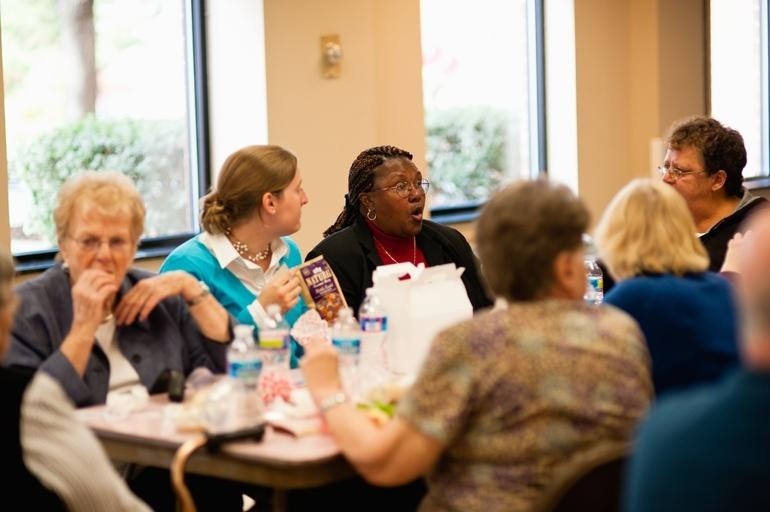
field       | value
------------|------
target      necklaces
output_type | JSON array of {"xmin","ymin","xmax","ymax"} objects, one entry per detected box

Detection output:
[
  {"xmin": 372, "ymin": 234, "xmax": 418, "ymax": 270},
  {"xmin": 227, "ymin": 233, "xmax": 272, "ymax": 267},
  {"xmin": 100, "ymin": 313, "xmax": 114, "ymax": 322}
]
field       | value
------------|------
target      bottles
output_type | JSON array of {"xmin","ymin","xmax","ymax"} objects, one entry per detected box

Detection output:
[
  {"xmin": 226, "ymin": 324, "xmax": 261, "ymax": 388},
  {"xmin": 358, "ymin": 287, "xmax": 388, "ymax": 372},
  {"xmin": 331, "ymin": 308, "xmax": 361, "ymax": 383},
  {"xmin": 258, "ymin": 303, "xmax": 289, "ymax": 376}
]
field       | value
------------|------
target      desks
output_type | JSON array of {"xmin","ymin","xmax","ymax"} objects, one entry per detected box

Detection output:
[{"xmin": 75, "ymin": 350, "xmax": 427, "ymax": 512}]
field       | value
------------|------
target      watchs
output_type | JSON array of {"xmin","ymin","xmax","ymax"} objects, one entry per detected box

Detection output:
[
  {"xmin": 318, "ymin": 392, "xmax": 348, "ymax": 412},
  {"xmin": 185, "ymin": 279, "xmax": 210, "ymax": 307}
]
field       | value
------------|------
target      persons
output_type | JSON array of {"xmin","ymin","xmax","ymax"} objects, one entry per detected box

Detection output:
[
  {"xmin": 157, "ymin": 144, "xmax": 309, "ymax": 372},
  {"xmin": 591, "ymin": 176, "xmax": 740, "ymax": 401},
  {"xmin": 302, "ymin": 144, "xmax": 495, "ymax": 320},
  {"xmin": 628, "ymin": 207, "xmax": 770, "ymax": 512},
  {"xmin": 0, "ymin": 251, "xmax": 157, "ymax": 512},
  {"xmin": 660, "ymin": 113, "xmax": 766, "ymax": 295},
  {"xmin": 293, "ymin": 173, "xmax": 656, "ymax": 512},
  {"xmin": 8, "ymin": 169, "xmax": 242, "ymax": 408}
]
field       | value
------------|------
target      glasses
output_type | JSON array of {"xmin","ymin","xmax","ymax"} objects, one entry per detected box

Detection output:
[
  {"xmin": 372, "ymin": 177, "xmax": 430, "ymax": 198},
  {"xmin": 659, "ymin": 164, "xmax": 705, "ymax": 180},
  {"xmin": 68, "ymin": 232, "xmax": 128, "ymax": 252}
]
